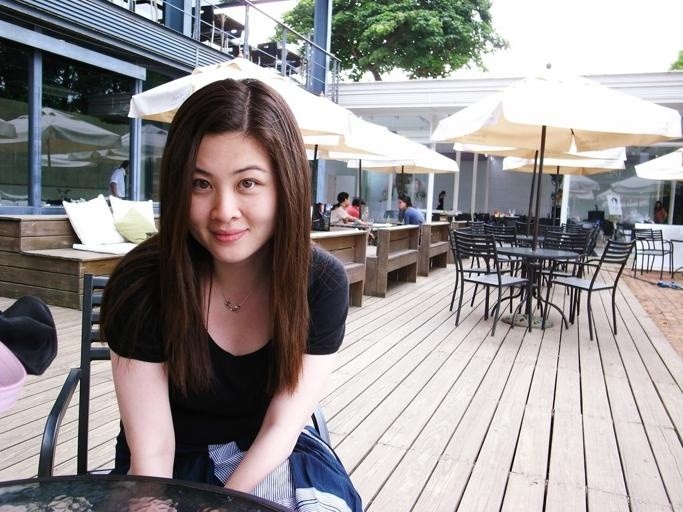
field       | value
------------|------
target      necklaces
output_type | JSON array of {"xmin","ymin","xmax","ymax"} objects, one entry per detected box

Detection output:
[{"xmin": 210, "ymin": 262, "xmax": 267, "ymax": 312}]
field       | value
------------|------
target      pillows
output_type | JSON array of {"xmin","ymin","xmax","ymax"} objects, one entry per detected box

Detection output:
[
  {"xmin": 114, "ymin": 210, "xmax": 158, "ymax": 244},
  {"xmin": 109, "ymin": 194, "xmax": 154, "ymax": 226},
  {"xmin": 61, "ymin": 193, "xmax": 129, "ymax": 244}
]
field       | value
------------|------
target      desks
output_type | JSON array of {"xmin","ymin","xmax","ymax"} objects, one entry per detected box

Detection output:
[
  {"xmin": 671, "ymin": 239, "xmax": 683, "ymax": 279},
  {"xmin": 0, "ymin": 472, "xmax": 303, "ymax": 512}
]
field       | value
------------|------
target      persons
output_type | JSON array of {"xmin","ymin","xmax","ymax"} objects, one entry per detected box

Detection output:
[
  {"xmin": 331, "ymin": 191, "xmax": 446, "ymax": 228},
  {"xmin": 310, "ymin": 203, "xmax": 331, "ymax": 231},
  {"xmin": 98, "ymin": 78, "xmax": 363, "ymax": 512},
  {"xmin": 107, "ymin": 159, "xmax": 130, "ymax": 200}
]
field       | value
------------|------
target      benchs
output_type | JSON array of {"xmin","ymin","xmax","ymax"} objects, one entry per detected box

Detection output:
[
  {"xmin": 2, "ymin": 213, "xmax": 163, "ymax": 311},
  {"xmin": 418, "ymin": 222, "xmax": 449, "ymax": 277},
  {"xmin": 363, "ymin": 224, "xmax": 419, "ymax": 299},
  {"xmin": 307, "ymin": 228, "xmax": 368, "ymax": 307}
]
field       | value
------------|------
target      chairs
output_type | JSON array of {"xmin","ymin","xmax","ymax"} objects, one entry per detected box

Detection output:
[
  {"xmin": 447, "ymin": 213, "xmax": 636, "ymax": 343},
  {"xmin": 631, "ymin": 228, "xmax": 674, "ymax": 280},
  {"xmin": 37, "ymin": 273, "xmax": 332, "ymax": 482}
]
